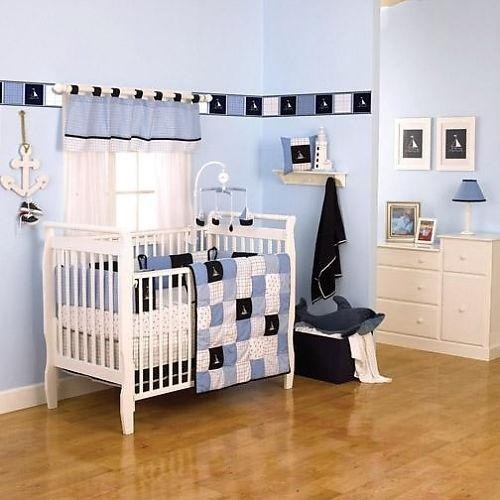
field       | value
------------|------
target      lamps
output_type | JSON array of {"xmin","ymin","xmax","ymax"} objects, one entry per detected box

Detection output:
[{"xmin": 451, "ymin": 180, "xmax": 486, "ymax": 235}]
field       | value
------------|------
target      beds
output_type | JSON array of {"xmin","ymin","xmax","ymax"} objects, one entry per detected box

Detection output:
[{"xmin": 42, "ymin": 209, "xmax": 296, "ymax": 435}]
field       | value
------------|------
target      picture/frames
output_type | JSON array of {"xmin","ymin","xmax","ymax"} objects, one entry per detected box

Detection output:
[
  {"xmin": 386, "ymin": 201, "xmax": 436, "ymax": 247},
  {"xmin": 395, "ymin": 118, "xmax": 477, "ymax": 171}
]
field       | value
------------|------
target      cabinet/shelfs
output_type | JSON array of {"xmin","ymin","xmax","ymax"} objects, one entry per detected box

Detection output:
[{"xmin": 374, "ymin": 233, "xmax": 499, "ymax": 361}]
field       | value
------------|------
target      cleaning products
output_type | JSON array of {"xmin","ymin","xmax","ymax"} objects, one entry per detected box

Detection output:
[{"xmin": 313, "ymin": 126, "xmax": 328, "ymax": 169}]
